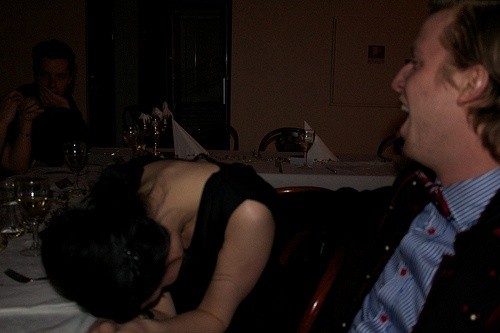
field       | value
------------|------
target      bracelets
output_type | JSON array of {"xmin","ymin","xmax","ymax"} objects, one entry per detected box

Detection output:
[{"xmin": 17, "ymin": 132, "xmax": 31, "ymax": 141}]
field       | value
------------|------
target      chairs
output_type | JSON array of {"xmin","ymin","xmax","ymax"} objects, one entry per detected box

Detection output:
[
  {"xmin": 268, "ymin": 229, "xmax": 344, "ymax": 333},
  {"xmin": 257, "ymin": 128, "xmax": 312, "ymax": 153}
]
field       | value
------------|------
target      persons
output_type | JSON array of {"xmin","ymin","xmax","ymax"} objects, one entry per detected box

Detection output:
[
  {"xmin": 280, "ymin": 0, "xmax": 500, "ymax": 333},
  {"xmin": 0, "ymin": 38, "xmax": 88, "ymax": 171},
  {"xmin": 39, "ymin": 151, "xmax": 322, "ymax": 333}
]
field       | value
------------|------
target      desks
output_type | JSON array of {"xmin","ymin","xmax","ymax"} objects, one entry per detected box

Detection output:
[
  {"xmin": 90, "ymin": 145, "xmax": 399, "ymax": 192},
  {"xmin": 0, "ymin": 238, "xmax": 103, "ymax": 333}
]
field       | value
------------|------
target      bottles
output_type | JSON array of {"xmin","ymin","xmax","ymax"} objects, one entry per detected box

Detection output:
[
  {"xmin": 132, "ymin": 113, "xmax": 150, "ymax": 159},
  {"xmin": 149, "ymin": 107, "xmax": 162, "ymax": 160},
  {"xmin": 159, "ymin": 101, "xmax": 175, "ymax": 160}
]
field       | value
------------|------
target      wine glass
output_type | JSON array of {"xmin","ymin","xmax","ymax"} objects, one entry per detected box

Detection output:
[
  {"xmin": 297, "ymin": 130, "xmax": 315, "ymax": 169},
  {"xmin": 0, "ymin": 177, "xmax": 25, "ymax": 234},
  {"xmin": 17, "ymin": 176, "xmax": 49, "ymax": 256},
  {"xmin": 64, "ymin": 142, "xmax": 86, "ymax": 196}
]
